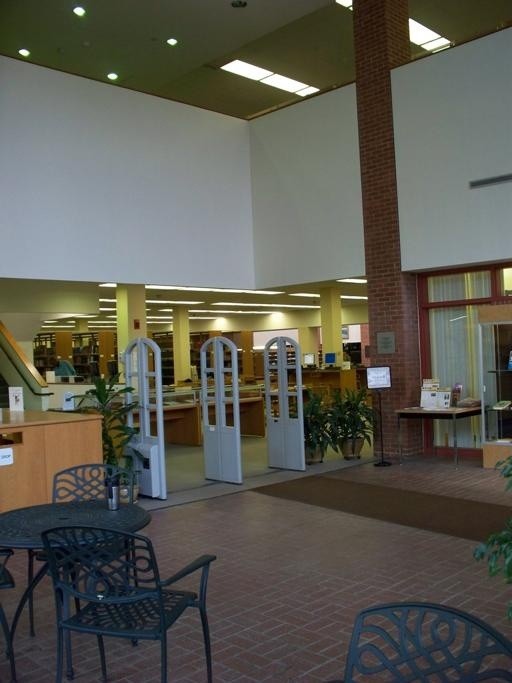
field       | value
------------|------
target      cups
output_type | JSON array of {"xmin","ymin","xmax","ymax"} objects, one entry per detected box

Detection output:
[{"xmin": 105, "ymin": 478, "xmax": 120, "ymax": 510}]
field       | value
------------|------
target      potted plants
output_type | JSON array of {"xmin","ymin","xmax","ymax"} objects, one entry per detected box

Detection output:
[
  {"xmin": 62, "ymin": 371, "xmax": 149, "ymax": 506},
  {"xmin": 289, "ymin": 383, "xmax": 380, "ymax": 464}
]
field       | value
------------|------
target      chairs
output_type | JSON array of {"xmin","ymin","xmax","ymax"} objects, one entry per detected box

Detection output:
[
  {"xmin": 40, "ymin": 526, "xmax": 216, "ymax": 683},
  {"xmin": 325, "ymin": 602, "xmax": 511, "ymax": 683},
  {"xmin": 1, "ymin": 547, "xmax": 17, "ymax": 682},
  {"xmin": 27, "ymin": 456, "xmax": 138, "ymax": 647}
]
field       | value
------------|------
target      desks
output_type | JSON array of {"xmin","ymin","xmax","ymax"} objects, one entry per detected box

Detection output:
[
  {"xmin": 1, "ymin": 499, "xmax": 151, "ymax": 683},
  {"xmin": 395, "ymin": 404, "xmax": 490, "ymax": 467}
]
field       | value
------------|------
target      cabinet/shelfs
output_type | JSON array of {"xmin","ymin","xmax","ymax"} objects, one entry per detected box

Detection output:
[
  {"xmin": 34, "ymin": 329, "xmax": 330, "ymax": 445},
  {"xmin": 477, "ymin": 303, "xmax": 512, "ymax": 469}
]
